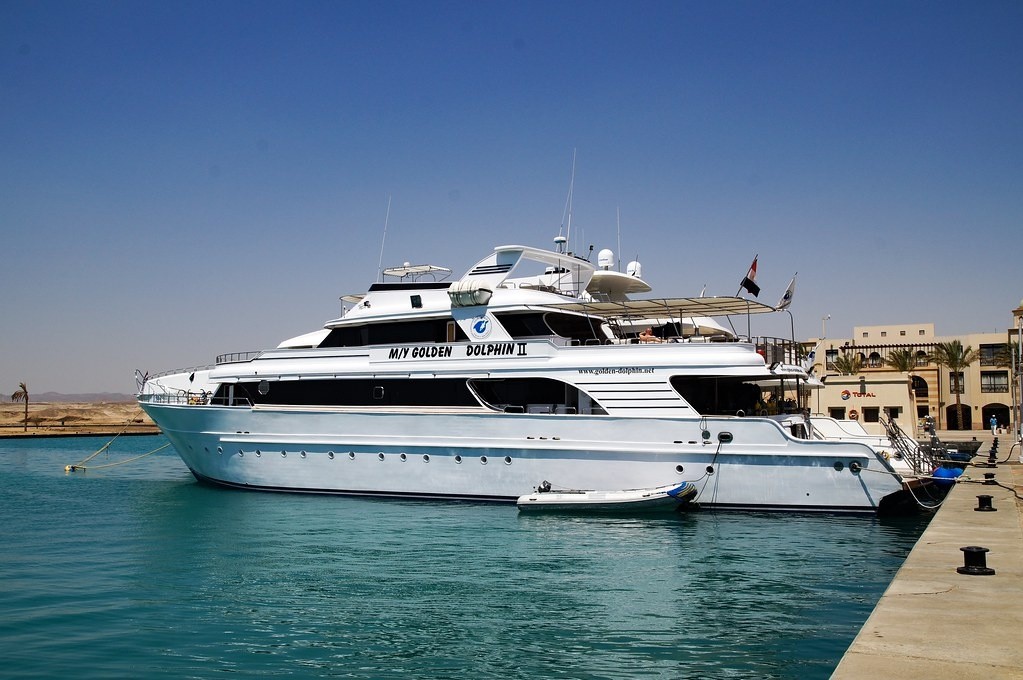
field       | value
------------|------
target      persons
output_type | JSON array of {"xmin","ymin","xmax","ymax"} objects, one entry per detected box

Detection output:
[
  {"xmin": 990, "ymin": 415, "xmax": 997, "ymax": 435},
  {"xmin": 731, "ymin": 382, "xmax": 798, "ymax": 415},
  {"xmin": 639, "ymin": 328, "xmax": 656, "ymax": 338}
]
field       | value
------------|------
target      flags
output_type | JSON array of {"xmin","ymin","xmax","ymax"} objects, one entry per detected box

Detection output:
[
  {"xmin": 741, "ymin": 259, "xmax": 760, "ymax": 298},
  {"xmin": 775, "ymin": 275, "xmax": 796, "ymax": 312}
]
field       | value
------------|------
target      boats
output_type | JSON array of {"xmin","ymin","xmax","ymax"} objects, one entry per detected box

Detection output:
[
  {"xmin": 516, "ymin": 478, "xmax": 697, "ymax": 513},
  {"xmin": 133, "ymin": 146, "xmax": 942, "ymax": 517}
]
